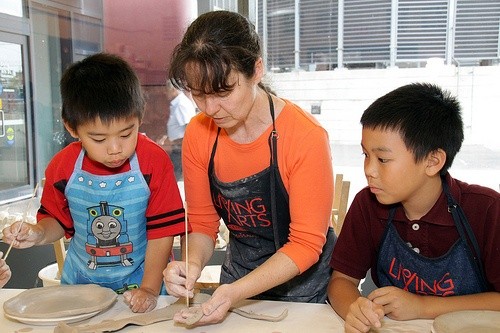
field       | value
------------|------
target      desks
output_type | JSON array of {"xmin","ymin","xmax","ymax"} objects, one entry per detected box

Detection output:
[{"xmin": 0, "ymin": 288, "xmax": 434, "ymax": 333}]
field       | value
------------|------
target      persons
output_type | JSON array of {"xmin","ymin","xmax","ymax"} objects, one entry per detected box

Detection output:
[
  {"xmin": 163, "ymin": 10, "xmax": 337, "ymax": 327},
  {"xmin": 2, "ymin": 50, "xmax": 192, "ymax": 314},
  {"xmin": 327, "ymin": 83, "xmax": 500, "ymax": 333},
  {"xmin": 164, "ymin": 78, "xmax": 196, "ymax": 181},
  {"xmin": 0, "ymin": 252, "xmax": 12, "ymax": 290}
]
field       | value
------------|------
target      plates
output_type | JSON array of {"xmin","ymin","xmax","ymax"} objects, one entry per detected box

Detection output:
[
  {"xmin": 5, "ymin": 311, "xmax": 100, "ymax": 325},
  {"xmin": 430, "ymin": 309, "xmax": 500, "ymax": 333}
]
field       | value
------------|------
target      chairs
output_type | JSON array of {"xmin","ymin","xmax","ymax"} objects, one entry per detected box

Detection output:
[{"xmin": 194, "ymin": 173, "xmax": 351, "ymax": 294}]
